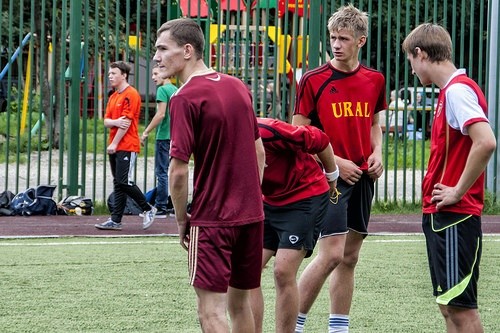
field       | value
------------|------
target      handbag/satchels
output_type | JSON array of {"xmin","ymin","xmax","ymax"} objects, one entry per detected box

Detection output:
[
  {"xmin": 0, "ymin": 190, "xmax": 17, "ymax": 216},
  {"xmin": 11, "ymin": 184, "xmax": 58, "ymax": 215}
]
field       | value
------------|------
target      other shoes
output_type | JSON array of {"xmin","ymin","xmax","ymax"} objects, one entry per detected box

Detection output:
[{"xmin": 139, "ymin": 205, "xmax": 167, "ymax": 218}]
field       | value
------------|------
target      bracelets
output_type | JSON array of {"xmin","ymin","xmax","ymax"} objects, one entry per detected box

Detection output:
[
  {"xmin": 143, "ymin": 132, "xmax": 148, "ymax": 137},
  {"xmin": 323, "ymin": 164, "xmax": 339, "ymax": 182}
]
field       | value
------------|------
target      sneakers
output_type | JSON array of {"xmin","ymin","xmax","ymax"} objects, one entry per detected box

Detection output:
[
  {"xmin": 143, "ymin": 204, "xmax": 158, "ymax": 230},
  {"xmin": 95, "ymin": 219, "xmax": 123, "ymax": 232}
]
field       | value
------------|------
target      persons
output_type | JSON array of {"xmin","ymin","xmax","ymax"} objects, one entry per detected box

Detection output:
[
  {"xmin": 292, "ymin": 4, "xmax": 387, "ymax": 333},
  {"xmin": 407, "ymin": 91, "xmax": 431, "ymax": 140},
  {"xmin": 254, "ymin": 116, "xmax": 340, "ymax": 333},
  {"xmin": 93, "ymin": 60, "xmax": 158, "ymax": 229},
  {"xmin": 386, "ymin": 89, "xmax": 404, "ymax": 127},
  {"xmin": 138, "ymin": 63, "xmax": 179, "ymax": 218},
  {"xmin": 403, "ymin": 23, "xmax": 496, "ymax": 333},
  {"xmin": 153, "ymin": 18, "xmax": 266, "ymax": 333}
]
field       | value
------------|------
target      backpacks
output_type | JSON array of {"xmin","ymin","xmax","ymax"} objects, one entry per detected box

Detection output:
[
  {"xmin": 58, "ymin": 196, "xmax": 95, "ymax": 215},
  {"xmin": 107, "ymin": 190, "xmax": 143, "ymax": 216}
]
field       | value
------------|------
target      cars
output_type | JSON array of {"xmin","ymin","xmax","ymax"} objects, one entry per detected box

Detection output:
[{"xmin": 399, "ymin": 87, "xmax": 442, "ymax": 108}]
